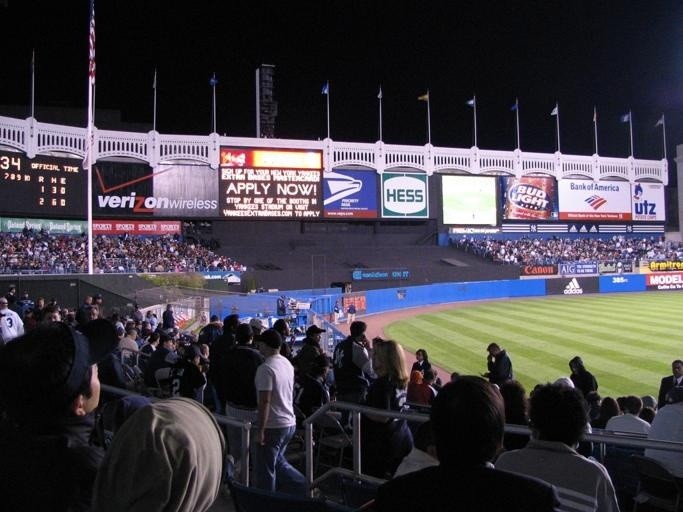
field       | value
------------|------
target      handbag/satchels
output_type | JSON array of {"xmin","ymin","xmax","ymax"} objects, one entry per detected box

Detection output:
[{"xmin": 372, "ymin": 419, "xmax": 413, "ymax": 468}]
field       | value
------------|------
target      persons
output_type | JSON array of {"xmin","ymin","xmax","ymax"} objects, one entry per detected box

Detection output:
[
  {"xmin": 2, "ymin": 284, "xmax": 408, "ymax": 511},
  {"xmin": 222, "ymin": 151, "xmax": 247, "ymax": 168},
  {"xmin": 457, "ymin": 232, "xmax": 683, "ymax": 266},
  {"xmin": 377, "ymin": 341, "xmax": 683, "ymax": 511},
  {"xmin": 1, "ymin": 227, "xmax": 246, "ymax": 275}
]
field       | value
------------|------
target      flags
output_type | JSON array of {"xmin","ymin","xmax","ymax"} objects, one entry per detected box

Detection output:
[
  {"xmin": 654, "ymin": 116, "xmax": 665, "ymax": 127},
  {"xmin": 466, "ymin": 98, "xmax": 475, "ymax": 107},
  {"xmin": 550, "ymin": 107, "xmax": 558, "ymax": 115},
  {"xmin": 418, "ymin": 94, "xmax": 428, "ymax": 101},
  {"xmin": 89, "ymin": 0, "xmax": 96, "ymax": 86},
  {"xmin": 618, "ymin": 112, "xmax": 632, "ymax": 123},
  {"xmin": 377, "ymin": 87, "xmax": 383, "ymax": 98},
  {"xmin": 321, "ymin": 82, "xmax": 329, "ymax": 95},
  {"xmin": 592, "ymin": 111, "xmax": 596, "ymax": 121},
  {"xmin": 511, "ymin": 102, "xmax": 518, "ymax": 110},
  {"xmin": 209, "ymin": 74, "xmax": 217, "ymax": 86}
]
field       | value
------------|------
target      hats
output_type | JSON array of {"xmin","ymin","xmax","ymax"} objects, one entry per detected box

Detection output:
[
  {"xmin": 306, "ymin": 325, "xmax": 326, "ymax": 335},
  {"xmin": 184, "ymin": 344, "xmax": 205, "ymax": 360},
  {"xmin": 148, "ymin": 333, "xmax": 160, "ymax": 343},
  {"xmin": 254, "ymin": 329, "xmax": 282, "ymax": 346},
  {"xmin": 0, "ymin": 319, "xmax": 118, "ymax": 418}
]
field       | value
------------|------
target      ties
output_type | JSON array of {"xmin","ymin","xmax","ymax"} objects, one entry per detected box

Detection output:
[{"xmin": 675, "ymin": 378, "xmax": 678, "ymax": 386}]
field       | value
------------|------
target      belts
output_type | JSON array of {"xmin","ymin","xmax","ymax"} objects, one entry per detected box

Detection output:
[{"xmin": 228, "ymin": 400, "xmax": 257, "ymax": 410}]
